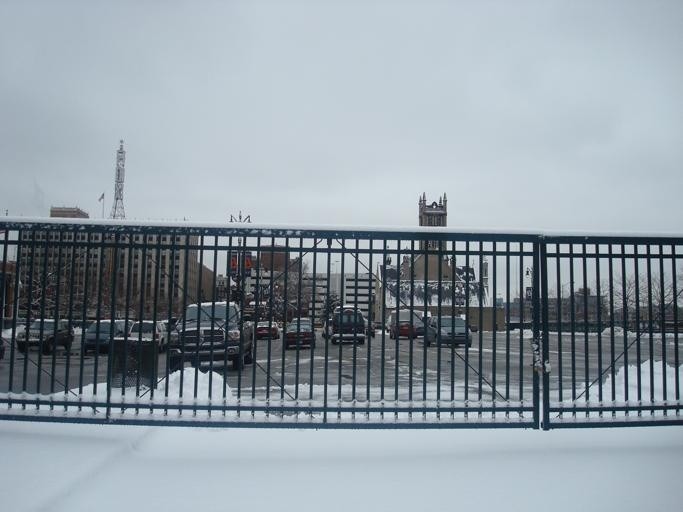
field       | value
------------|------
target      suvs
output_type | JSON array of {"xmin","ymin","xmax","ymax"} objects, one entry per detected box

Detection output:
[
  {"xmin": 390, "ymin": 310, "xmax": 416, "ymax": 340},
  {"xmin": 16, "ymin": 318, "xmax": 74, "ymax": 356},
  {"xmin": 169, "ymin": 301, "xmax": 253, "ymax": 373}
]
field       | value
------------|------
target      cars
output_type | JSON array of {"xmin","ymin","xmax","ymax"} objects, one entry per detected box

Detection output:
[
  {"xmin": 257, "ymin": 321, "xmax": 280, "ymax": 340},
  {"xmin": 424, "ymin": 316, "xmax": 472, "ymax": 347},
  {"xmin": 322, "ymin": 319, "xmax": 332, "ymax": 339},
  {"xmin": 416, "ymin": 321, "xmax": 426, "ymax": 338},
  {"xmin": 286, "ymin": 318, "xmax": 316, "ymax": 349},
  {"xmin": 85, "ymin": 320, "xmax": 172, "ymax": 355},
  {"xmin": 363, "ymin": 317, "xmax": 376, "ymax": 338}
]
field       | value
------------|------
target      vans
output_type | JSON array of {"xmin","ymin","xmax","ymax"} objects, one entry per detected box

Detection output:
[{"xmin": 332, "ymin": 305, "xmax": 365, "ymax": 344}]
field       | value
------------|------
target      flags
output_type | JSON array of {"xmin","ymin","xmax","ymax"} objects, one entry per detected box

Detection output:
[{"xmin": 98, "ymin": 193, "xmax": 103, "ymax": 201}]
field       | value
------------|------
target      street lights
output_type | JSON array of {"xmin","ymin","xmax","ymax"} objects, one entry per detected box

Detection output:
[{"xmin": 228, "ymin": 210, "xmax": 252, "ymax": 300}]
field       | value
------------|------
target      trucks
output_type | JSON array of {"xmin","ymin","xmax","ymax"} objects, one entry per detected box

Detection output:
[{"xmin": 387, "ymin": 309, "xmax": 431, "ymax": 331}]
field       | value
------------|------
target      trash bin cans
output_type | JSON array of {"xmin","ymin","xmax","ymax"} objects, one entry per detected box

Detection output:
[{"xmin": 110, "ymin": 338, "xmax": 157, "ymax": 389}]
